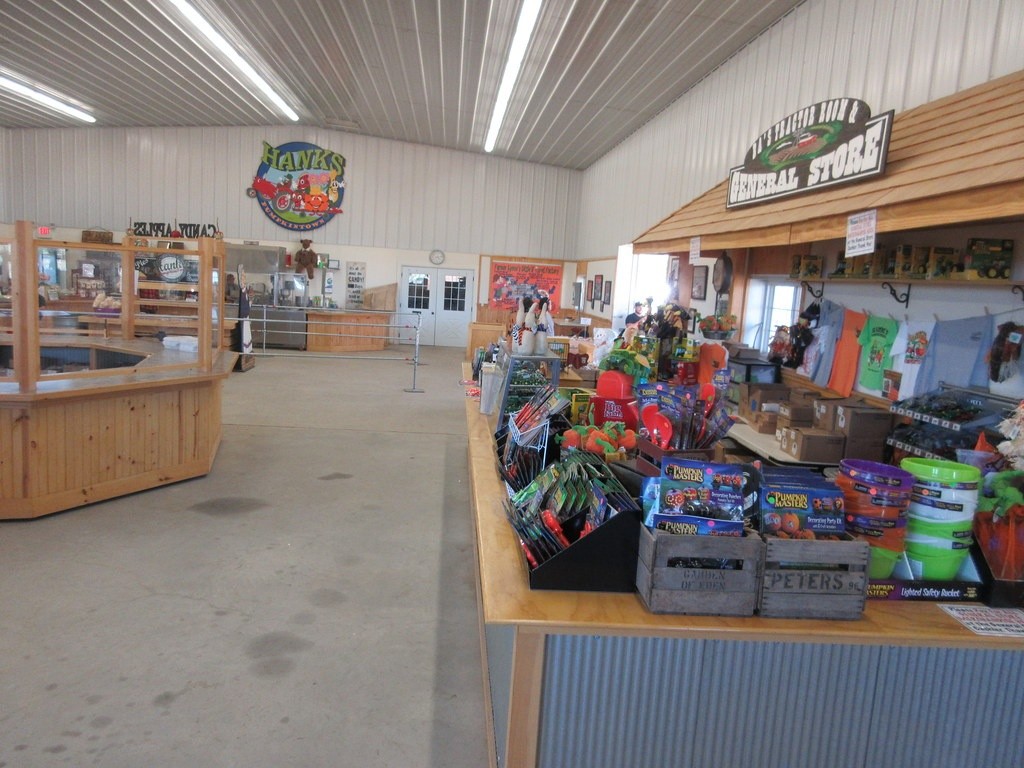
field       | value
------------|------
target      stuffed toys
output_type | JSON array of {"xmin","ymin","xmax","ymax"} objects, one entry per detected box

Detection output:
[{"xmin": 295, "ymin": 239, "xmax": 317, "ymax": 278}]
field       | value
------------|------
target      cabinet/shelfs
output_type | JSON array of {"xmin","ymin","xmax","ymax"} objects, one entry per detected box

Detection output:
[{"xmin": 490, "ymin": 343, "xmax": 562, "ymax": 434}]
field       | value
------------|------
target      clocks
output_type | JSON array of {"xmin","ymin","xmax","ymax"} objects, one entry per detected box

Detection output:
[
  {"xmin": 711, "ymin": 254, "xmax": 733, "ymax": 293},
  {"xmin": 430, "ymin": 250, "xmax": 445, "ymax": 264}
]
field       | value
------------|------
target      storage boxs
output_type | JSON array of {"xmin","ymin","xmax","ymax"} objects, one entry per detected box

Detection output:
[
  {"xmin": 670, "ymin": 338, "xmax": 701, "ymax": 362},
  {"xmin": 557, "ymin": 386, "xmax": 596, "ymax": 426},
  {"xmin": 726, "ymin": 359, "xmax": 894, "ymax": 463},
  {"xmin": 522, "ymin": 452, "xmax": 643, "ymax": 592},
  {"xmin": 866, "ymin": 539, "xmax": 1023, "ymax": 607},
  {"xmin": 94, "ymin": 307, "xmax": 121, "ymax": 319},
  {"xmin": 636, "ymin": 437, "xmax": 714, "ymax": 479},
  {"xmin": 788, "ymin": 254, "xmax": 823, "ymax": 281},
  {"xmin": 635, "ymin": 495, "xmax": 870, "ymax": 619},
  {"xmin": 826, "ymin": 236, "xmax": 1014, "ymax": 282},
  {"xmin": 633, "ymin": 335, "xmax": 661, "ymax": 381}
]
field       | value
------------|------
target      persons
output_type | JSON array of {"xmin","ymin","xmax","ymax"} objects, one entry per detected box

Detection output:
[
  {"xmin": 224, "ymin": 274, "xmax": 239, "ymax": 302},
  {"xmin": 626, "ymin": 302, "xmax": 646, "ymax": 326}
]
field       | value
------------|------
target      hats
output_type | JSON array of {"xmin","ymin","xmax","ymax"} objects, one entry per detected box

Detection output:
[{"xmin": 635, "ymin": 302, "xmax": 642, "ymax": 307}]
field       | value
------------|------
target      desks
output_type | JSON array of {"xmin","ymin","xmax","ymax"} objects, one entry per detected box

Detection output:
[
  {"xmin": 554, "ymin": 318, "xmax": 587, "ymax": 338},
  {"xmin": 724, "ymin": 402, "xmax": 800, "ymax": 467},
  {"xmin": 461, "ymin": 364, "xmax": 1024, "ymax": 768}
]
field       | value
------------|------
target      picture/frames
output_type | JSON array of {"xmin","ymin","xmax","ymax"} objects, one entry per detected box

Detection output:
[
  {"xmin": 691, "ymin": 265, "xmax": 708, "ymax": 300},
  {"xmin": 594, "ymin": 275, "xmax": 603, "ymax": 300},
  {"xmin": 316, "ymin": 253, "xmax": 329, "ymax": 267},
  {"xmin": 328, "ymin": 260, "xmax": 339, "ymax": 269},
  {"xmin": 587, "ymin": 281, "xmax": 593, "ymax": 302},
  {"xmin": 604, "ymin": 281, "xmax": 611, "ymax": 305}
]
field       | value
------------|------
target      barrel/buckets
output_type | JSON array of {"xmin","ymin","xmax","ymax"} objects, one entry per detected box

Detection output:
[{"xmin": 835, "ymin": 453, "xmax": 980, "ymax": 584}]
